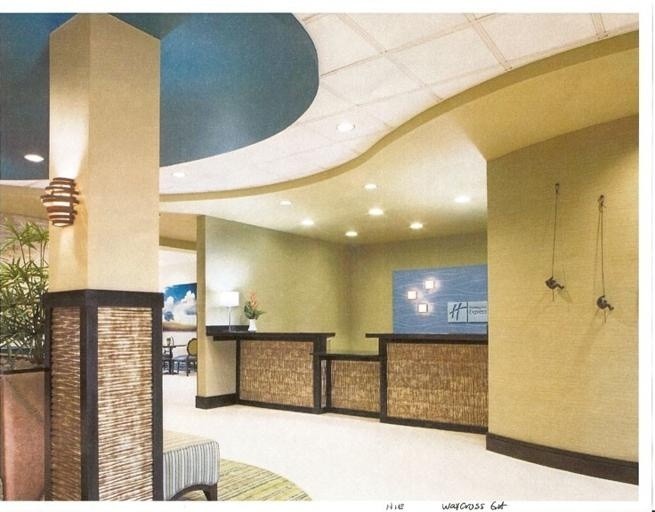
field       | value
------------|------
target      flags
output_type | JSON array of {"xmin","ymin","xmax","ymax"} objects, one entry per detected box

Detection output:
[{"xmin": 246, "ymin": 318, "xmax": 258, "ymax": 332}]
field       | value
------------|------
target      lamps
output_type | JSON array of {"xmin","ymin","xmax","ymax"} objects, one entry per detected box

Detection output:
[{"xmin": 161, "ymin": 338, "xmax": 197, "ymax": 378}]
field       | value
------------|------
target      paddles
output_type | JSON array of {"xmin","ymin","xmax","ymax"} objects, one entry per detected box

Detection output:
[{"xmin": 168, "ymin": 455, "xmax": 317, "ymax": 503}]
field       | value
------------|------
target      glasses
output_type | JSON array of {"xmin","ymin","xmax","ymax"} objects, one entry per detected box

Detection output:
[{"xmin": 38, "ymin": 176, "xmax": 81, "ymax": 229}]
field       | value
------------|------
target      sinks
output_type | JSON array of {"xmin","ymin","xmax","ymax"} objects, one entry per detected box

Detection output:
[{"xmin": 162, "ymin": 424, "xmax": 218, "ymax": 501}]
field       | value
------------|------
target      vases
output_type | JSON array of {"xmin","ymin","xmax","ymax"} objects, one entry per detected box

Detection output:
[{"xmin": 243, "ymin": 290, "xmax": 267, "ymax": 319}]
